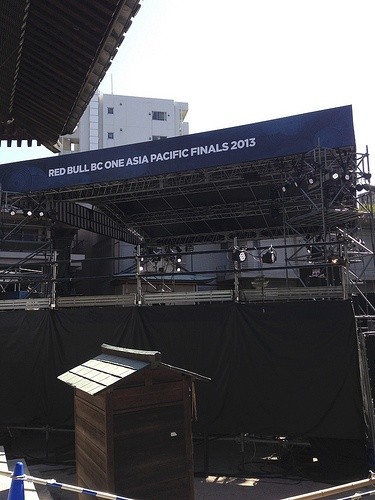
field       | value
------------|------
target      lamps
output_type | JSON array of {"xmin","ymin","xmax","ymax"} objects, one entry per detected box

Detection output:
[
  {"xmin": 34, "ymin": 212, "xmax": 44, "ymax": 217},
  {"xmin": 140, "ymin": 266, "xmax": 144, "ymax": 270},
  {"xmin": 23, "ymin": 211, "xmax": 32, "ymax": 216},
  {"xmin": 282, "ymin": 184, "xmax": 288, "ymax": 192},
  {"xmin": 9, "ymin": 210, "xmax": 16, "ymax": 216},
  {"xmin": 177, "ymin": 267, "xmax": 181, "ymax": 272},
  {"xmin": 294, "ymin": 182, "xmax": 301, "ymax": 187},
  {"xmin": 177, "ymin": 257, "xmax": 182, "ymax": 263},
  {"xmin": 308, "ymin": 177, "xmax": 317, "ymax": 184},
  {"xmin": 342, "ymin": 174, "xmax": 350, "ymax": 180},
  {"xmin": 330, "ymin": 172, "xmax": 339, "ymax": 179},
  {"xmin": 233, "ymin": 251, "xmax": 246, "ymax": 262},
  {"xmin": 262, "ymin": 253, "xmax": 275, "ymax": 263}
]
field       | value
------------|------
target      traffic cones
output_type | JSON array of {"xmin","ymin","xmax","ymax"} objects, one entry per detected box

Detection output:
[{"xmin": 7, "ymin": 462, "xmax": 25, "ymax": 500}]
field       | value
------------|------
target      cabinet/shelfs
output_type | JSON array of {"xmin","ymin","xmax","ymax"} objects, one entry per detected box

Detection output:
[{"xmin": 57, "ymin": 343, "xmax": 213, "ymax": 500}]
